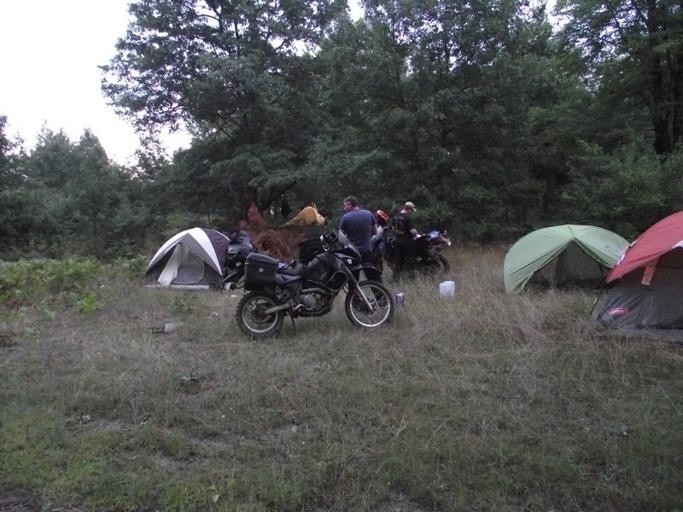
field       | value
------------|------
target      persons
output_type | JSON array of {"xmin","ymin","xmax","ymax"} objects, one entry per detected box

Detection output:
[
  {"xmin": 386, "ymin": 201, "xmax": 424, "ymax": 284},
  {"xmin": 336, "ymin": 195, "xmax": 387, "ymax": 312}
]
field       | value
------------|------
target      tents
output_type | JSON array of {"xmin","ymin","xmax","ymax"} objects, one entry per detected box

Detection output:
[
  {"xmin": 145, "ymin": 226, "xmax": 232, "ymax": 289},
  {"xmin": 581, "ymin": 207, "xmax": 683, "ymax": 329},
  {"xmin": 503, "ymin": 224, "xmax": 630, "ymax": 308}
]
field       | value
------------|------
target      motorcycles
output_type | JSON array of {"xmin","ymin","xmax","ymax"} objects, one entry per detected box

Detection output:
[{"xmin": 236, "ymin": 230, "xmax": 394, "ymax": 339}]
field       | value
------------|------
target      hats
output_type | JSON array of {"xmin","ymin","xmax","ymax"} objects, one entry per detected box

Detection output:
[{"xmin": 405, "ymin": 201, "xmax": 418, "ymax": 213}]
